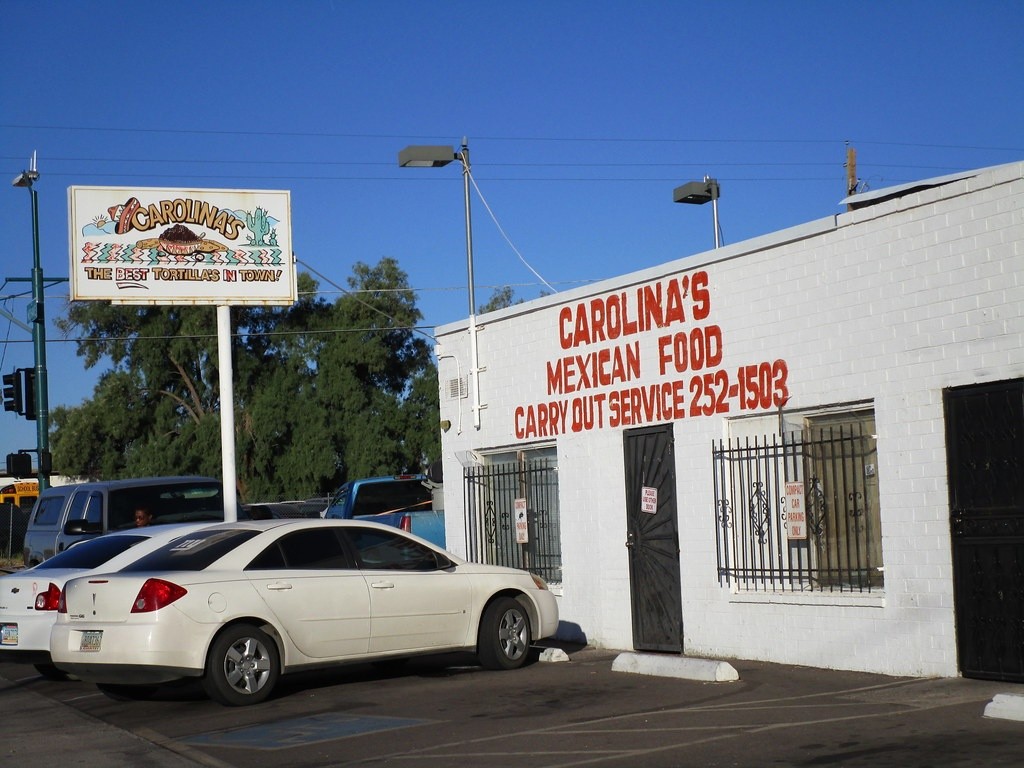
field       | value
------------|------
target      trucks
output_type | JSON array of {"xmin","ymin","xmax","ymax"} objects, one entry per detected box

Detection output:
[{"xmin": 324, "ymin": 473, "xmax": 446, "ymax": 552}]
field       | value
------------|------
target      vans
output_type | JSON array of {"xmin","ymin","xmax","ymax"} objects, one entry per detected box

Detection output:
[{"xmin": 23, "ymin": 474, "xmax": 247, "ymax": 569}]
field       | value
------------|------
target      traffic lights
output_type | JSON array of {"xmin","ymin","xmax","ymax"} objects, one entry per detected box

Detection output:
[
  {"xmin": 2, "ymin": 370, "xmax": 24, "ymax": 412},
  {"xmin": 7, "ymin": 452, "xmax": 32, "ymax": 478}
]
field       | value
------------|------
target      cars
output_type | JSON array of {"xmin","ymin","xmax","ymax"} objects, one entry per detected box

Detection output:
[
  {"xmin": 48, "ymin": 517, "xmax": 560, "ymax": 707},
  {"xmin": 241, "ymin": 497, "xmax": 334, "ymax": 519},
  {"xmin": 0, "ymin": 522, "xmax": 228, "ymax": 681}
]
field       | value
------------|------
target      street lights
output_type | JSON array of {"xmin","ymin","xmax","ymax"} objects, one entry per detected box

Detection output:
[
  {"xmin": 673, "ymin": 175, "xmax": 721, "ymax": 249},
  {"xmin": 13, "ymin": 149, "xmax": 52, "ymax": 493},
  {"xmin": 397, "ymin": 137, "xmax": 482, "ymax": 428}
]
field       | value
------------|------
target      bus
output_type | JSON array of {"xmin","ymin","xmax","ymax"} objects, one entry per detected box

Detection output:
[{"xmin": 0, "ymin": 482, "xmax": 53, "ymax": 516}]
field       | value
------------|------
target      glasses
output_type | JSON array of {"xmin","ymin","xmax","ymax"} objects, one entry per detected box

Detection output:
[{"xmin": 135, "ymin": 515, "xmax": 146, "ymax": 520}]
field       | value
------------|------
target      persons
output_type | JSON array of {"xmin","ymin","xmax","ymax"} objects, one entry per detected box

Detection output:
[{"xmin": 134, "ymin": 504, "xmax": 153, "ymax": 529}]
